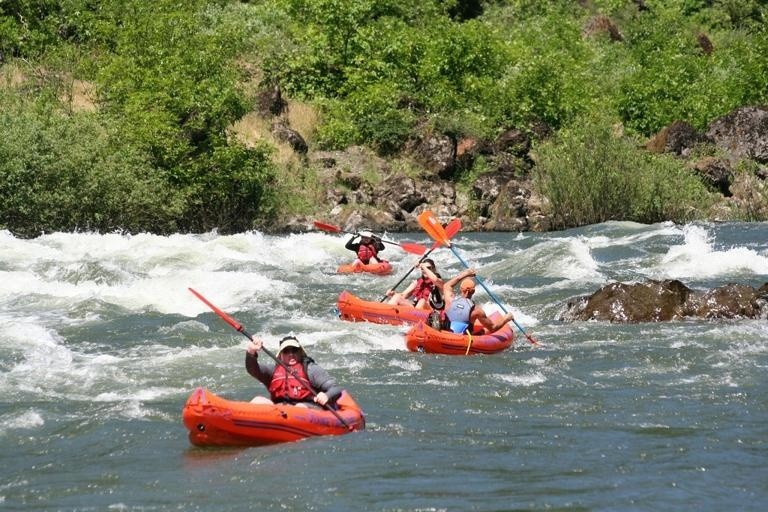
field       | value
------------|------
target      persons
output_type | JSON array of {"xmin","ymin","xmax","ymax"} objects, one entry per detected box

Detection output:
[
  {"xmin": 442, "ymin": 267, "xmax": 515, "ymax": 335},
  {"xmin": 384, "ymin": 260, "xmax": 445, "ymax": 311},
  {"xmin": 245, "ymin": 331, "xmax": 342, "ymax": 411},
  {"xmin": 344, "ymin": 226, "xmax": 386, "ymax": 267}
]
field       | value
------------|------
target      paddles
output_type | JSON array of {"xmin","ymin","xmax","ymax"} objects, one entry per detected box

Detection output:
[
  {"xmin": 188, "ymin": 285, "xmax": 355, "ymax": 431},
  {"xmin": 416, "ymin": 210, "xmax": 536, "ymax": 346},
  {"xmin": 313, "ymin": 219, "xmax": 425, "ymax": 256},
  {"xmin": 381, "ymin": 218, "xmax": 461, "ymax": 304}
]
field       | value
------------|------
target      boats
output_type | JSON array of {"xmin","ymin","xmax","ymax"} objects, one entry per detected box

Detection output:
[
  {"xmin": 405, "ymin": 308, "xmax": 520, "ymax": 356},
  {"xmin": 180, "ymin": 381, "xmax": 372, "ymax": 450},
  {"xmin": 334, "ymin": 260, "xmax": 397, "ymax": 282},
  {"xmin": 334, "ymin": 285, "xmax": 452, "ymax": 334}
]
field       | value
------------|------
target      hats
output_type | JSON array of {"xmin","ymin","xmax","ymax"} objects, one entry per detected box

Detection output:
[
  {"xmin": 276, "ymin": 340, "xmax": 300, "ymax": 358},
  {"xmin": 460, "ymin": 279, "xmax": 475, "ymax": 293}
]
